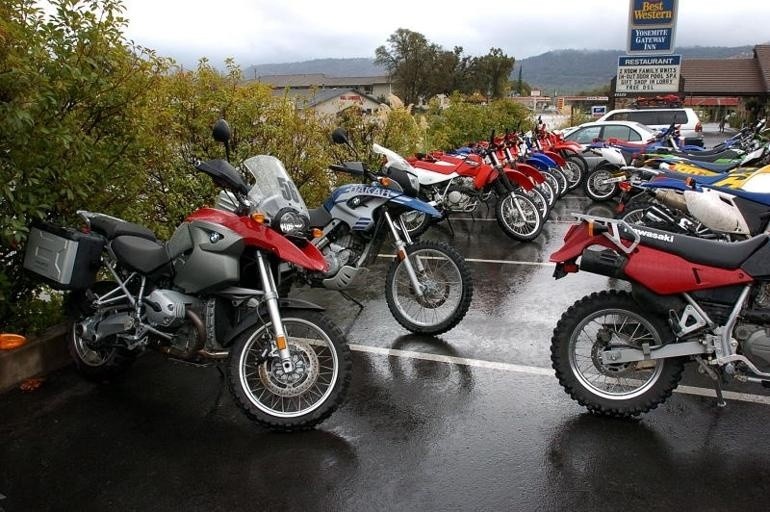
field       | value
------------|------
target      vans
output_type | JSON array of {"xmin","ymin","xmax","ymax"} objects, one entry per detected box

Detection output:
[{"xmin": 596, "ymin": 108, "xmax": 704, "ymax": 148}]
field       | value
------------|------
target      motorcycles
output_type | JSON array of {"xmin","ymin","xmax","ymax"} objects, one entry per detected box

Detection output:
[
  {"xmin": 215, "ymin": 128, "xmax": 474, "ymax": 335},
  {"xmin": 393, "ymin": 115, "xmax": 588, "ymax": 241},
  {"xmin": 24, "ymin": 119, "xmax": 352, "ymax": 431}
]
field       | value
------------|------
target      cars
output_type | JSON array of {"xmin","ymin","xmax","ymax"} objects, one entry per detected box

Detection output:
[{"xmin": 563, "ymin": 120, "xmax": 657, "ymax": 173}]
